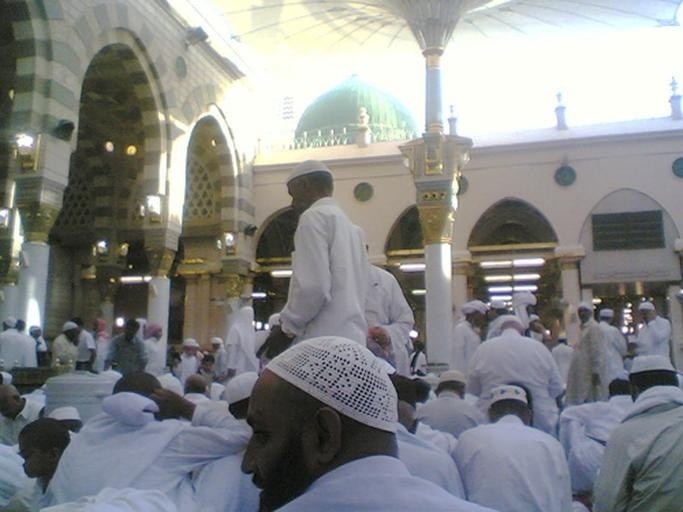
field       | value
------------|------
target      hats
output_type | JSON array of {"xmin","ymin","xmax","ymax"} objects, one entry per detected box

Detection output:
[
  {"xmin": 577, "ymin": 300, "xmax": 595, "ymax": 312},
  {"xmin": 434, "ymin": 370, "xmax": 467, "ymax": 394},
  {"xmin": 46, "ymin": 406, "xmax": 82, "ymax": 422},
  {"xmin": 490, "ymin": 299, "xmax": 505, "ymax": 309},
  {"xmin": 489, "ymin": 385, "xmax": 529, "ymax": 407},
  {"xmin": 183, "ymin": 338, "xmax": 197, "ymax": 347},
  {"xmin": 630, "ymin": 353, "xmax": 677, "ymax": 375},
  {"xmin": 3, "ymin": 317, "xmax": 18, "ymax": 329},
  {"xmin": 357, "ymin": 222, "xmax": 370, "ymax": 247},
  {"xmin": 265, "ymin": 335, "xmax": 401, "ymax": 434},
  {"xmin": 196, "ymin": 342, "xmax": 200, "ymax": 348},
  {"xmin": 1, "ymin": 370, "xmax": 13, "ymax": 386},
  {"xmin": 598, "ymin": 307, "xmax": 616, "ymax": 318},
  {"xmin": 284, "ymin": 159, "xmax": 332, "ymax": 184},
  {"xmin": 269, "ymin": 313, "xmax": 283, "ymax": 330},
  {"xmin": 638, "ymin": 301, "xmax": 656, "ymax": 312},
  {"xmin": 225, "ymin": 371, "xmax": 260, "ymax": 405},
  {"xmin": 557, "ymin": 329, "xmax": 568, "ymax": 340},
  {"xmin": 29, "ymin": 326, "xmax": 40, "ymax": 333},
  {"xmin": 62, "ymin": 321, "xmax": 78, "ymax": 333}
]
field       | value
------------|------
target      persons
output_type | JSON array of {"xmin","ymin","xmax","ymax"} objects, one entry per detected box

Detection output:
[
  {"xmin": 525, "ymin": 313, "xmax": 549, "ymax": 346},
  {"xmin": 256, "ymin": 159, "xmax": 368, "ymax": 359},
  {"xmin": 551, "ymin": 331, "xmax": 573, "ymax": 382},
  {"xmin": 573, "ymin": 302, "xmax": 596, "ymax": 342},
  {"xmin": 211, "ymin": 337, "xmax": 226, "ymax": 378},
  {"xmin": 513, "ymin": 292, "xmax": 536, "ymax": 332},
  {"xmin": 174, "ymin": 337, "xmax": 200, "ymax": 385},
  {"xmin": 389, "ymin": 372, "xmax": 464, "ymax": 495},
  {"xmin": 141, "ymin": 322, "xmax": 165, "ymax": 374},
  {"xmin": 223, "ymin": 368, "xmax": 260, "ymax": 418},
  {"xmin": 635, "ymin": 299, "xmax": 671, "ymax": 359},
  {"xmin": 409, "ymin": 342, "xmax": 426, "ymax": 371},
  {"xmin": 182, "ymin": 374, "xmax": 208, "ymax": 402},
  {"xmin": 0, "ymin": 383, "xmax": 44, "ymax": 446},
  {"xmin": 198, "ymin": 354, "xmax": 224, "ymax": 391},
  {"xmin": 241, "ymin": 335, "xmax": 497, "ymax": 512},
  {"xmin": 16, "ymin": 319, "xmax": 37, "ymax": 367},
  {"xmin": 558, "ymin": 368, "xmax": 631, "ymax": 493},
  {"xmin": 104, "ymin": 317, "xmax": 149, "ymax": 375},
  {"xmin": 364, "ymin": 239, "xmax": 414, "ymax": 375},
  {"xmin": 488, "ymin": 300, "xmax": 507, "ymax": 319},
  {"xmin": 29, "ymin": 326, "xmax": 48, "ymax": 366},
  {"xmin": 454, "ymin": 384, "xmax": 589, "ymax": 512},
  {"xmin": 47, "ymin": 370, "xmax": 250, "ymax": 512},
  {"xmin": 48, "ymin": 406, "xmax": 82, "ymax": 433},
  {"xmin": 417, "ymin": 370, "xmax": 486, "ymax": 436},
  {"xmin": 598, "ymin": 309, "xmax": 626, "ymax": 371},
  {"xmin": 0, "ymin": 317, "xmax": 24, "ymax": 371},
  {"xmin": 51, "ymin": 321, "xmax": 79, "ymax": 372},
  {"xmin": 468, "ymin": 315, "xmax": 567, "ymax": 437},
  {"xmin": 590, "ymin": 354, "xmax": 682, "ymax": 511},
  {"xmin": 71, "ymin": 317, "xmax": 97, "ymax": 371},
  {"xmin": 452, "ymin": 299, "xmax": 488, "ymax": 375},
  {"xmin": 0, "ymin": 416, "xmax": 69, "ymax": 512}
]
